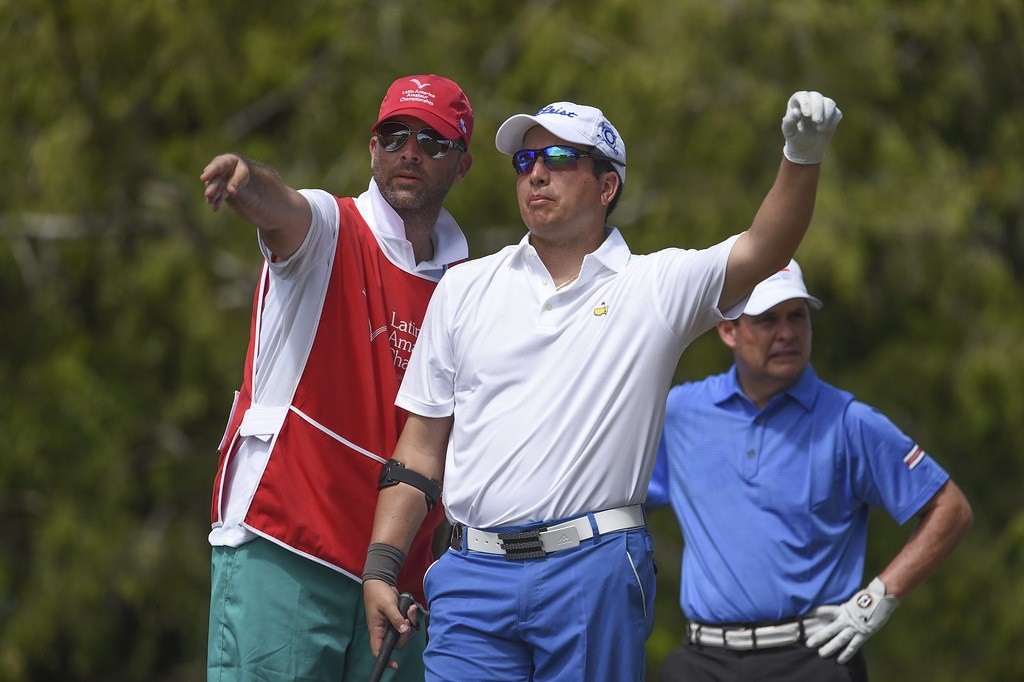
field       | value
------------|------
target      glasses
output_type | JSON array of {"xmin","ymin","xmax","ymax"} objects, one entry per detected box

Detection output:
[
  {"xmin": 511, "ymin": 146, "xmax": 626, "ymax": 176},
  {"xmin": 376, "ymin": 122, "xmax": 466, "ymax": 160}
]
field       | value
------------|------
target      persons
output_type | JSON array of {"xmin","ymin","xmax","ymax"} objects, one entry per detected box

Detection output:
[
  {"xmin": 361, "ymin": 91, "xmax": 845, "ymax": 682},
  {"xmin": 202, "ymin": 75, "xmax": 471, "ymax": 682},
  {"xmin": 641, "ymin": 259, "xmax": 971, "ymax": 682}
]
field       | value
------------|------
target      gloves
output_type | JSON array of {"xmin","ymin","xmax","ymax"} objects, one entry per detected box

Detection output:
[
  {"xmin": 781, "ymin": 90, "xmax": 842, "ymax": 165},
  {"xmin": 804, "ymin": 576, "xmax": 901, "ymax": 663}
]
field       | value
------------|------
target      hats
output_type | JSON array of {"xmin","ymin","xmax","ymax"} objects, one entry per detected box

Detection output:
[
  {"xmin": 496, "ymin": 101, "xmax": 626, "ymax": 184},
  {"xmin": 744, "ymin": 257, "xmax": 824, "ymax": 316},
  {"xmin": 370, "ymin": 75, "xmax": 473, "ymax": 150}
]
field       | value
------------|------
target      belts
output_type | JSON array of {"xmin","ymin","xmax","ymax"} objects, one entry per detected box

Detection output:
[
  {"xmin": 449, "ymin": 504, "xmax": 645, "ymax": 558},
  {"xmin": 685, "ymin": 620, "xmax": 828, "ymax": 651}
]
